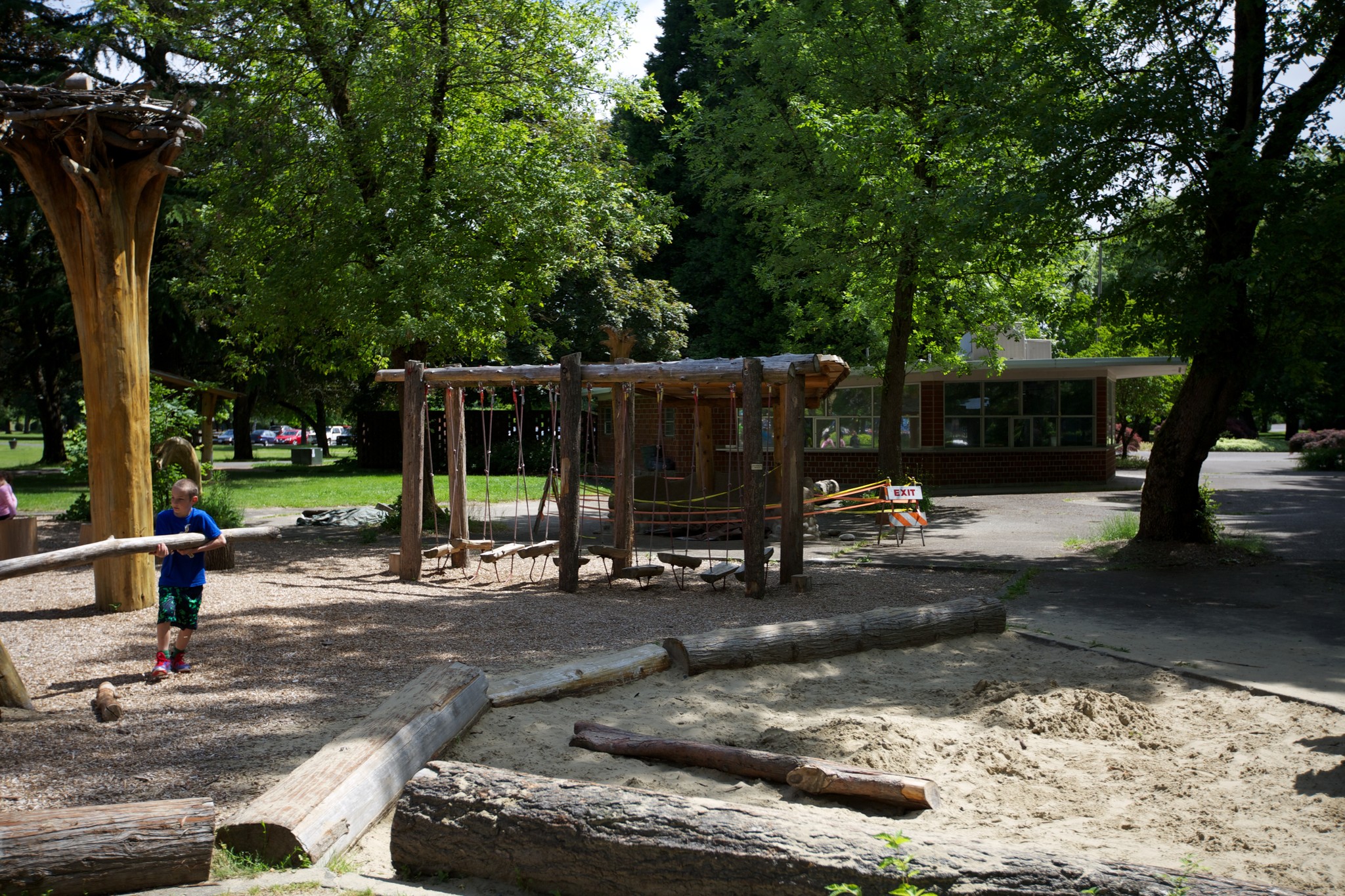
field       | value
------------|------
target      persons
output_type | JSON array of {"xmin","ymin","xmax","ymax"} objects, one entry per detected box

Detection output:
[
  {"xmin": 0, "ymin": 473, "xmax": 17, "ymax": 519},
  {"xmin": 761, "ymin": 414, "xmax": 912, "ymax": 449},
  {"xmin": 148, "ymin": 478, "xmax": 227, "ymax": 679}
]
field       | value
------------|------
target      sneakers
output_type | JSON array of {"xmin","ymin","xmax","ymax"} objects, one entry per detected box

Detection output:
[
  {"xmin": 151, "ymin": 651, "xmax": 172, "ymax": 677},
  {"xmin": 170, "ymin": 651, "xmax": 192, "ymax": 672}
]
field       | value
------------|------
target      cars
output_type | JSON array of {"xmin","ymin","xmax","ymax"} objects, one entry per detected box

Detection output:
[
  {"xmin": 342, "ymin": 425, "xmax": 352, "ymax": 430},
  {"xmin": 250, "ymin": 430, "xmax": 277, "ymax": 446},
  {"xmin": 737, "ymin": 408, "xmax": 774, "ymax": 449},
  {"xmin": 840, "ymin": 418, "xmax": 910, "ymax": 438},
  {"xmin": 217, "ymin": 429, "xmax": 235, "ymax": 445},
  {"xmin": 274, "ymin": 429, "xmax": 311, "ymax": 446}
]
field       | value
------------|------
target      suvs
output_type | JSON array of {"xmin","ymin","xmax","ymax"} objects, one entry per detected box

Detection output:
[
  {"xmin": 336, "ymin": 429, "xmax": 356, "ymax": 446},
  {"xmin": 269, "ymin": 425, "xmax": 293, "ymax": 436},
  {"xmin": 307, "ymin": 426, "xmax": 345, "ymax": 446}
]
